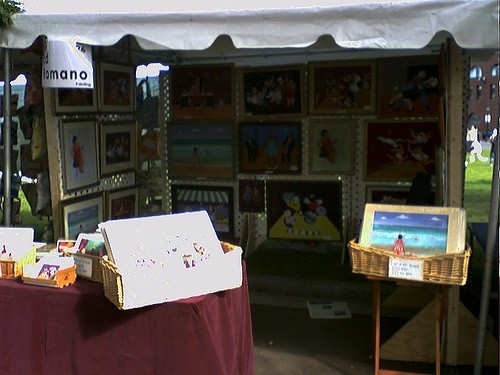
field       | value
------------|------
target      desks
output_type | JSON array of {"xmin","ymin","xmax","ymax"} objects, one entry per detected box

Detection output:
[
  {"xmin": 368, "ymin": 274, "xmax": 455, "ymax": 375},
  {"xmin": 0, "ymin": 261, "xmax": 254, "ymax": 375}
]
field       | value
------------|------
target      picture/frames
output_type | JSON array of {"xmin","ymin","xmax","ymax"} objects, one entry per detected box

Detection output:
[
  {"xmin": 32, "ymin": 33, "xmax": 140, "ymax": 279},
  {"xmin": 157, "ymin": 46, "xmax": 464, "ymax": 265}
]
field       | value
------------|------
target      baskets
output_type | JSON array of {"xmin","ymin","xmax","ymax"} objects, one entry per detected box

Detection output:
[
  {"xmin": 70, "ymin": 242, "xmax": 242, "ymax": 309},
  {"xmin": 0, "ymin": 246, "xmax": 37, "ymax": 279},
  {"xmin": 348, "ymin": 237, "xmax": 472, "ymax": 286}
]
